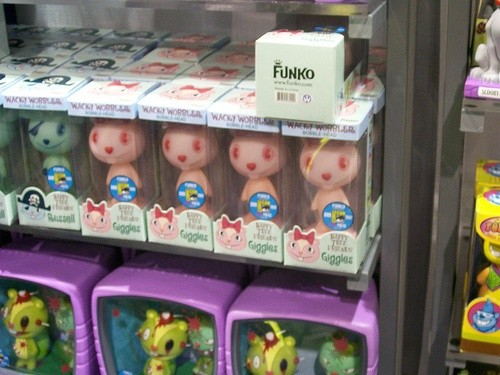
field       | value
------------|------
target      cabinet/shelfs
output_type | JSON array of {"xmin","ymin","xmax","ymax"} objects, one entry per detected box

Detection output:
[
  {"xmin": 1, "ymin": 0, "xmax": 412, "ymax": 375},
  {"xmin": 411, "ymin": 2, "xmax": 500, "ymax": 375}
]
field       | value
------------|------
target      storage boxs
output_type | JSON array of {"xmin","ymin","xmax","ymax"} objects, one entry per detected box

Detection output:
[
  {"xmin": 253, "ymin": 17, "xmax": 372, "ymax": 126},
  {"xmin": 459, "ymin": 158, "xmax": 500, "ymax": 360},
  {"xmin": 0, "ymin": 24, "xmax": 386, "ymax": 372}
]
